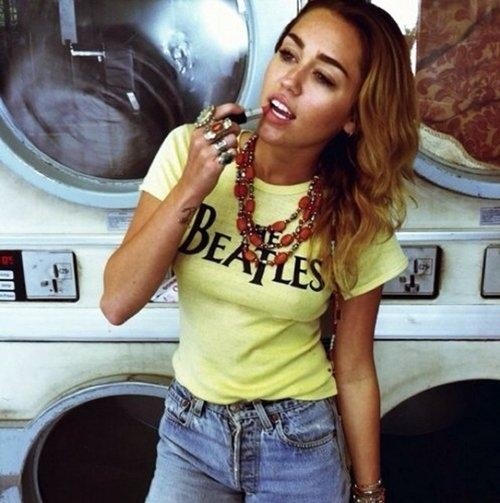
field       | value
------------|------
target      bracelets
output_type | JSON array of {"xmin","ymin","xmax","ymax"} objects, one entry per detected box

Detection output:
[{"xmin": 352, "ymin": 478, "xmax": 386, "ymax": 503}]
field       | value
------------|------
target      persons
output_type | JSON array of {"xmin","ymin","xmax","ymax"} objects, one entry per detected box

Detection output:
[{"xmin": 98, "ymin": 0, "xmax": 422, "ymax": 502}]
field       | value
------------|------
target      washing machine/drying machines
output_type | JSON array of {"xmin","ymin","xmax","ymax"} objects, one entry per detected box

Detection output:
[
  {"xmin": 0, "ymin": 0, "xmax": 500, "ymax": 342},
  {"xmin": 0, "ymin": 337, "xmax": 499, "ymax": 503}
]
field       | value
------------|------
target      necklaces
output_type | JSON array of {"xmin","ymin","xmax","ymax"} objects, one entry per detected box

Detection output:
[{"xmin": 232, "ymin": 132, "xmax": 340, "ymax": 365}]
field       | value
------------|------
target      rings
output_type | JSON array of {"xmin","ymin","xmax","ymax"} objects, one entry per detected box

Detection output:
[
  {"xmin": 193, "ymin": 103, "xmax": 215, "ymax": 128},
  {"xmin": 210, "ymin": 137, "xmax": 228, "ymax": 155},
  {"xmin": 202, "ymin": 117, "xmax": 233, "ymax": 143},
  {"xmin": 217, "ymin": 151, "xmax": 233, "ymax": 165}
]
